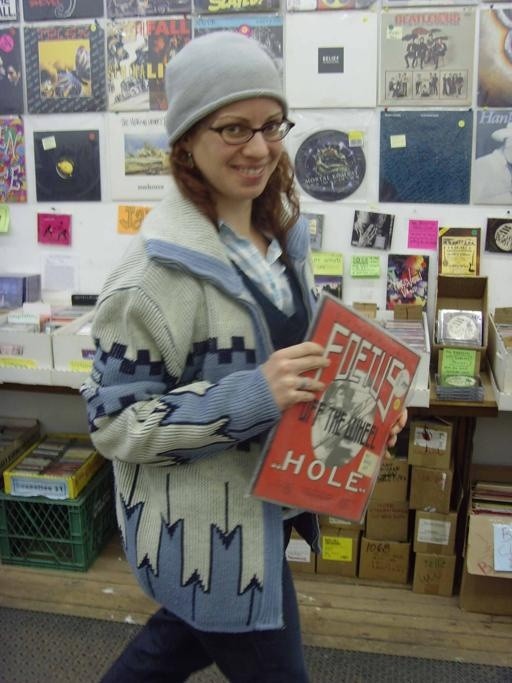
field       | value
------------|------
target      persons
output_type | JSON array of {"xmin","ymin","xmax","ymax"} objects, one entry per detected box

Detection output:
[
  {"xmin": 351, "ymin": 211, "xmax": 391, "ymax": 249},
  {"xmin": 387, "ymin": 28, "xmax": 465, "ymax": 101},
  {"xmin": 107, "ymin": 21, "xmax": 192, "ymax": 100},
  {"xmin": 0, "ymin": 60, "xmax": 24, "ymax": 114},
  {"xmin": 79, "ymin": 30, "xmax": 409, "ymax": 683}
]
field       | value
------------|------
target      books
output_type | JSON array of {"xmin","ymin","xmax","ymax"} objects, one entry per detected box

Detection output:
[
  {"xmin": 471, "ymin": 479, "xmax": 512, "ymax": 520},
  {"xmin": 241, "ymin": 291, "xmax": 422, "ymax": 525}
]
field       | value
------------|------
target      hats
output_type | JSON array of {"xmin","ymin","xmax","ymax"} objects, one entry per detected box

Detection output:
[{"xmin": 165, "ymin": 31, "xmax": 288, "ymax": 147}]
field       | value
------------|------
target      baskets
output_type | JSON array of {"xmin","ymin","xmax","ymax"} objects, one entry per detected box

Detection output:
[{"xmin": 0, "ymin": 461, "xmax": 118, "ymax": 572}]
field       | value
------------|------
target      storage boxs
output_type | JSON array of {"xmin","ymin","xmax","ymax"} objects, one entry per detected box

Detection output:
[
  {"xmin": 285, "ymin": 413, "xmax": 458, "ymax": 599},
  {"xmin": 486, "ymin": 305, "xmax": 512, "ymax": 412},
  {"xmin": 430, "ymin": 271, "xmax": 489, "ymax": 353},
  {"xmin": 0, "ymin": 299, "xmax": 101, "ymax": 373},
  {"xmin": 456, "ymin": 464, "xmax": 512, "ymax": 618}
]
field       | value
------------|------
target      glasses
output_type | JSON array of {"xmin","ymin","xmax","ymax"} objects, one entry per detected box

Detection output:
[{"xmin": 200, "ymin": 118, "xmax": 295, "ymax": 146}]
